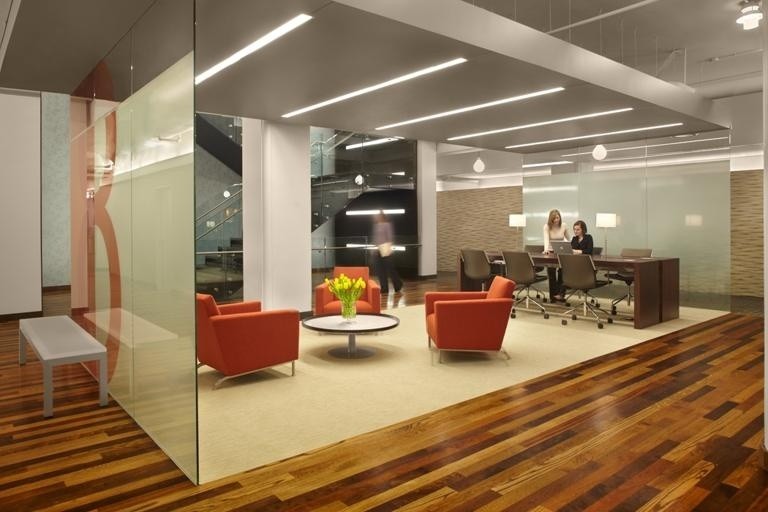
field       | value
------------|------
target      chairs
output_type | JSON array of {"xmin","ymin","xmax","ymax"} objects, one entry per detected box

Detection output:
[
  {"xmin": 314, "ymin": 266, "xmax": 381, "ymax": 316},
  {"xmin": 456, "ymin": 244, "xmax": 681, "ymax": 331},
  {"xmin": 425, "ymin": 274, "xmax": 516, "ymax": 363},
  {"xmin": 196, "ymin": 294, "xmax": 300, "ymax": 388}
]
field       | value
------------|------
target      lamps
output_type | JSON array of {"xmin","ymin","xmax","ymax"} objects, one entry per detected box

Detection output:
[
  {"xmin": 596, "ymin": 212, "xmax": 617, "ymax": 256},
  {"xmin": 735, "ymin": 0, "xmax": 763, "ymax": 30},
  {"xmin": 508, "ymin": 213, "xmax": 528, "ymax": 250}
]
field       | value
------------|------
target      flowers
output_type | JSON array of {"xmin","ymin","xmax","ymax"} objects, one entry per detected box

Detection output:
[{"xmin": 323, "ymin": 273, "xmax": 366, "ymax": 319}]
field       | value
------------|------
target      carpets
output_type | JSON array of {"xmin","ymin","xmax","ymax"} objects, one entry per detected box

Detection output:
[{"xmin": 197, "ymin": 303, "xmax": 731, "ymax": 484}]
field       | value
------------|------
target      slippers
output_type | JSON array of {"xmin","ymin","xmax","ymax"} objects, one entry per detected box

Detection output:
[{"xmin": 552, "ymin": 296, "xmax": 566, "ymax": 302}]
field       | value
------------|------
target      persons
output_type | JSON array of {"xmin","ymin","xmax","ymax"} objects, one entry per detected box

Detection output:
[
  {"xmin": 541, "ymin": 209, "xmax": 571, "ymax": 304},
  {"xmin": 552, "ymin": 220, "xmax": 593, "ymax": 300},
  {"xmin": 372, "ymin": 209, "xmax": 402, "ymax": 297}
]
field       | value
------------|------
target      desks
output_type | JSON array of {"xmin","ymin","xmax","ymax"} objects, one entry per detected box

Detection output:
[{"xmin": 303, "ymin": 314, "xmax": 400, "ymax": 362}]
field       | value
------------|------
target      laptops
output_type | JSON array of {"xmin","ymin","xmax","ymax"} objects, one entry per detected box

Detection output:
[{"xmin": 551, "ymin": 241, "xmax": 574, "ymax": 256}]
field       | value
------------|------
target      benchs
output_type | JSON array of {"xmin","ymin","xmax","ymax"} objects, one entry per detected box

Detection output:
[{"xmin": 17, "ymin": 316, "xmax": 109, "ymax": 416}]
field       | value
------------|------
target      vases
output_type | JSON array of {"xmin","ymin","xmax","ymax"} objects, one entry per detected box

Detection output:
[{"xmin": 340, "ymin": 300, "xmax": 356, "ymax": 323}]
